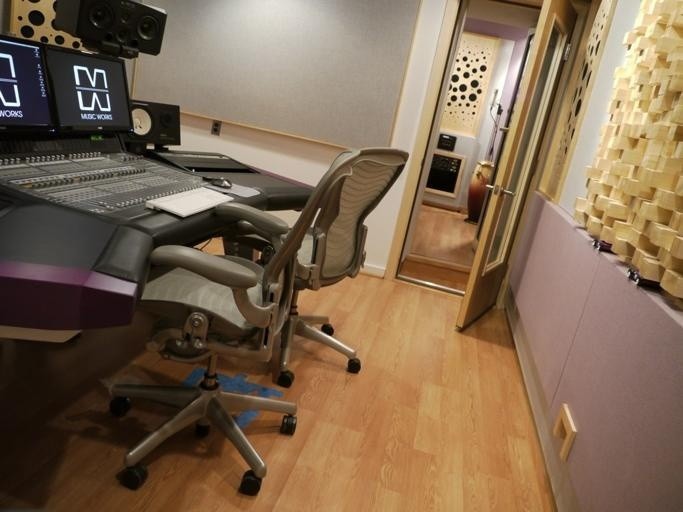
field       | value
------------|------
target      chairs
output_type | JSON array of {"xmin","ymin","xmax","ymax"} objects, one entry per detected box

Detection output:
[
  {"xmin": 161, "ymin": 148, "xmax": 408, "ymax": 388},
  {"xmin": 109, "ymin": 150, "xmax": 359, "ymax": 495}
]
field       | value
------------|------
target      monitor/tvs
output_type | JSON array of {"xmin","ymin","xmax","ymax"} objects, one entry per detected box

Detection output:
[
  {"xmin": 44, "ymin": 43, "xmax": 133, "ymax": 136},
  {"xmin": 0, "ymin": 33, "xmax": 57, "ymax": 132}
]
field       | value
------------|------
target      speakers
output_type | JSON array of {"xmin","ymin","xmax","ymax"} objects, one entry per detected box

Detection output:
[
  {"xmin": 123, "ymin": 98, "xmax": 181, "ymax": 146},
  {"xmin": 55, "ymin": 0, "xmax": 167, "ymax": 60}
]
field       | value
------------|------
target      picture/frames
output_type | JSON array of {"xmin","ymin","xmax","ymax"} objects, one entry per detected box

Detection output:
[{"xmin": 424, "ymin": 149, "xmax": 468, "ymax": 199}]
